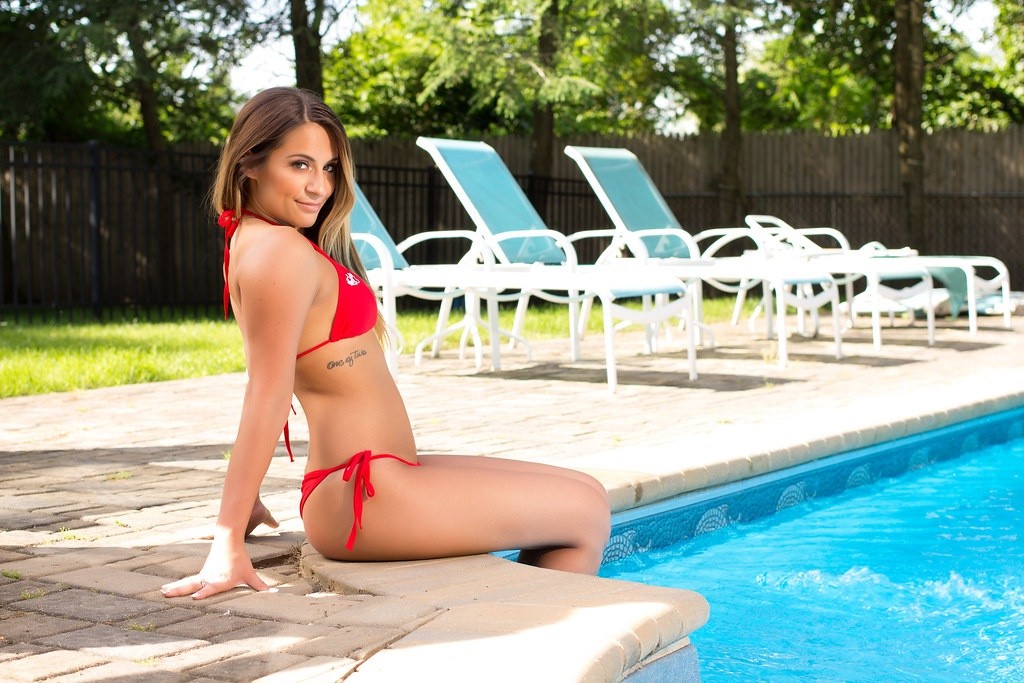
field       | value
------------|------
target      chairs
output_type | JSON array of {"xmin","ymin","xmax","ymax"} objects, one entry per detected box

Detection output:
[{"xmin": 347, "ymin": 136, "xmax": 1024, "ymax": 381}]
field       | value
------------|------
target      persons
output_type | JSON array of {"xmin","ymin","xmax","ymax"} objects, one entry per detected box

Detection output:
[{"xmin": 162, "ymin": 86, "xmax": 611, "ymax": 599}]
